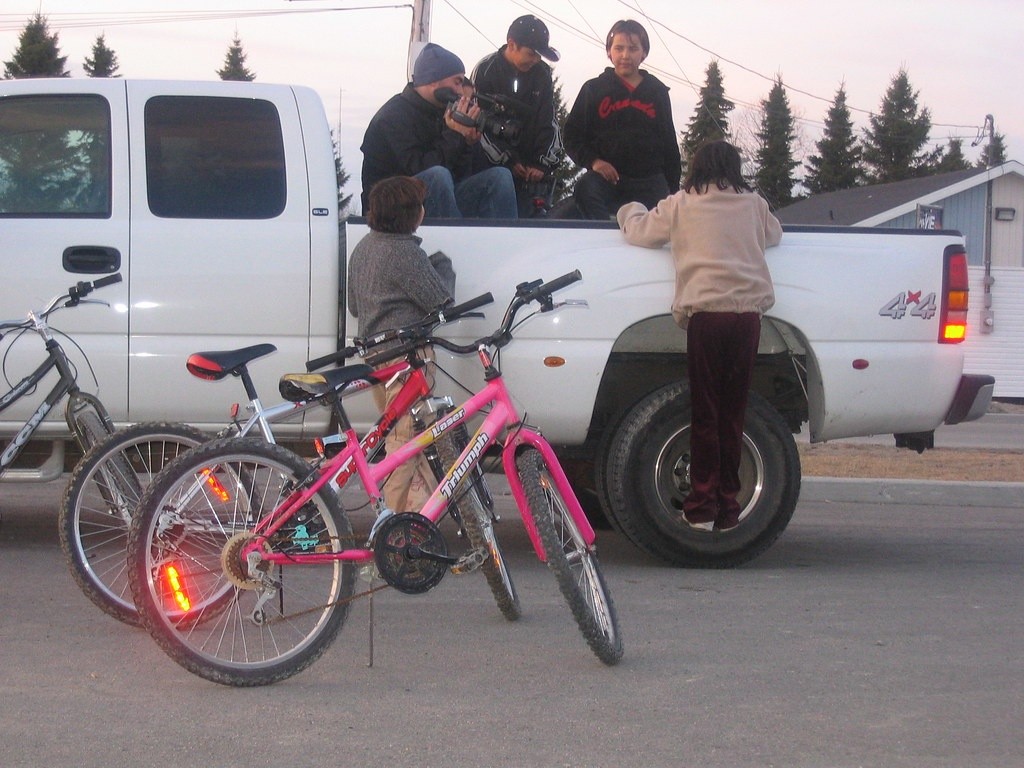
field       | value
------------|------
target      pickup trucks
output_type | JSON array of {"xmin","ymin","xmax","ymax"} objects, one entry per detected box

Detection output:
[{"xmin": 0, "ymin": 75, "xmax": 994, "ymax": 573}]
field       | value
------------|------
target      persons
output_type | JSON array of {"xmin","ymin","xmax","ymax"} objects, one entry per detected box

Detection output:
[
  {"xmin": 347, "ymin": 175, "xmax": 455, "ymax": 577},
  {"xmin": 359, "ymin": 43, "xmax": 519, "ymax": 218},
  {"xmin": 615, "ymin": 139, "xmax": 782, "ymax": 532},
  {"xmin": 469, "ymin": 14, "xmax": 562, "ymax": 217},
  {"xmin": 563, "ymin": 20, "xmax": 682, "ymax": 220}
]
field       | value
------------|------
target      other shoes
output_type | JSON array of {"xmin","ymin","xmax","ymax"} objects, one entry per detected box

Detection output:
[{"xmin": 682, "ymin": 511, "xmax": 714, "ymax": 532}]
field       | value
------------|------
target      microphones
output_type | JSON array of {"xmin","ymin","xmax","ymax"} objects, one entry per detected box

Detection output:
[{"xmin": 487, "ymin": 94, "xmax": 536, "ymax": 120}]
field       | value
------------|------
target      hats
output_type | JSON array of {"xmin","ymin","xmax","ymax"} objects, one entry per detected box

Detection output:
[
  {"xmin": 413, "ymin": 43, "xmax": 465, "ymax": 87},
  {"xmin": 510, "ymin": 15, "xmax": 559, "ymax": 61}
]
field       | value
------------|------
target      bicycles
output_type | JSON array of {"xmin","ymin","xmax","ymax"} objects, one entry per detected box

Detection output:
[
  {"xmin": 0, "ymin": 270, "xmax": 143, "ymax": 539},
  {"xmin": 58, "ymin": 291, "xmax": 523, "ymax": 633},
  {"xmin": 124, "ymin": 263, "xmax": 626, "ymax": 689}
]
